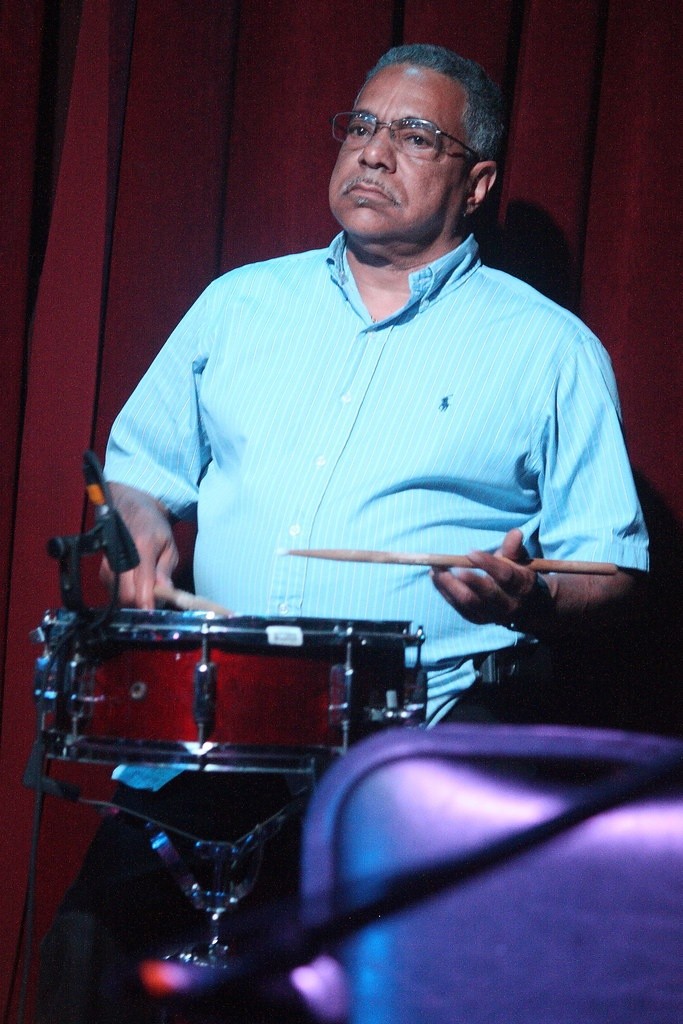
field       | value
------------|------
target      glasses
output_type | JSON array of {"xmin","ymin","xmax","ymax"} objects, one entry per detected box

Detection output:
[{"xmin": 331, "ymin": 112, "xmax": 479, "ymax": 160}]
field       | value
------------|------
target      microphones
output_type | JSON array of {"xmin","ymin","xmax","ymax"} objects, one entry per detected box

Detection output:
[{"xmin": 82, "ymin": 459, "xmax": 141, "ymax": 573}]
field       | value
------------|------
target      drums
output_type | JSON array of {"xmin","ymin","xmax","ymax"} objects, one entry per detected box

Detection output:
[{"xmin": 42, "ymin": 606, "xmax": 412, "ymax": 781}]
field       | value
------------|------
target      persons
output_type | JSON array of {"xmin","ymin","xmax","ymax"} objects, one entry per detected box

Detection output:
[{"xmin": 32, "ymin": 44, "xmax": 649, "ymax": 1023}]
